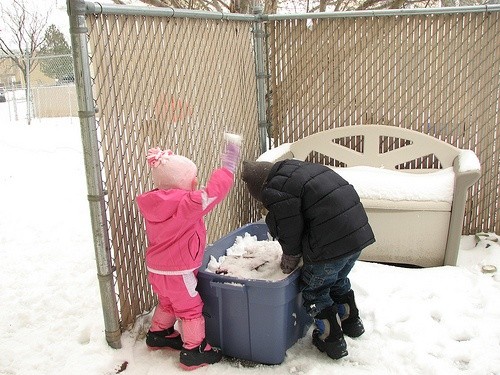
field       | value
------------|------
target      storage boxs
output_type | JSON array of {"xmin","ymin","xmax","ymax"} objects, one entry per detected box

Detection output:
[{"xmin": 195, "ymin": 217, "xmax": 313, "ymax": 366}]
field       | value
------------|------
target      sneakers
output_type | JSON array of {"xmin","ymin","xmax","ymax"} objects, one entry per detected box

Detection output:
[
  {"xmin": 311, "ymin": 328, "xmax": 349, "ymax": 360},
  {"xmin": 178, "ymin": 339, "xmax": 223, "ymax": 371},
  {"xmin": 144, "ymin": 326, "xmax": 183, "ymax": 352},
  {"xmin": 343, "ymin": 319, "xmax": 366, "ymax": 338}
]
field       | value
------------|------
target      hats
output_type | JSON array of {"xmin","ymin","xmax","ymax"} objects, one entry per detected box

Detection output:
[
  {"xmin": 146, "ymin": 146, "xmax": 198, "ymax": 191},
  {"xmin": 239, "ymin": 159, "xmax": 275, "ymax": 202}
]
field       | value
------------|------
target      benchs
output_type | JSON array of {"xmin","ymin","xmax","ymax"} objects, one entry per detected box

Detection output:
[{"xmin": 254, "ymin": 124, "xmax": 483, "ymax": 269}]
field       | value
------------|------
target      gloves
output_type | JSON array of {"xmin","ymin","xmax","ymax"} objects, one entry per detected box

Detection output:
[
  {"xmin": 220, "ymin": 133, "xmax": 243, "ymax": 175},
  {"xmin": 280, "ymin": 253, "xmax": 301, "ymax": 275}
]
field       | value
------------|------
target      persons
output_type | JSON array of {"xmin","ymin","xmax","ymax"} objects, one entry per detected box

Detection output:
[
  {"xmin": 134, "ymin": 133, "xmax": 243, "ymax": 370},
  {"xmin": 240, "ymin": 159, "xmax": 376, "ymax": 360}
]
